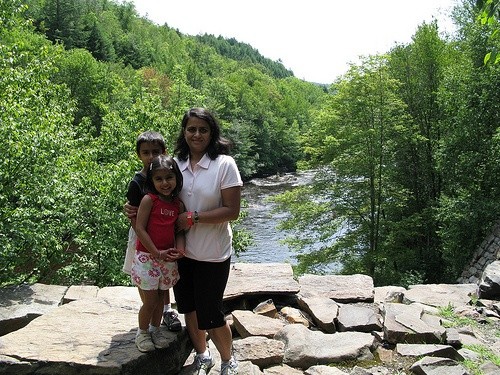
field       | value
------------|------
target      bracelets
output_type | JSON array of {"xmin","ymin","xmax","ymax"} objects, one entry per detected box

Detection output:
[
  {"xmin": 195, "ymin": 210, "xmax": 200, "ymax": 223},
  {"xmin": 187, "ymin": 211, "xmax": 193, "ymax": 225}
]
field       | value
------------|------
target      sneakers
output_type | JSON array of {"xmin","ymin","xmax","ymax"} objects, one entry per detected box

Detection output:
[
  {"xmin": 190, "ymin": 350, "xmax": 215, "ymax": 375},
  {"xmin": 221, "ymin": 356, "xmax": 238, "ymax": 375},
  {"xmin": 135, "ymin": 331, "xmax": 155, "ymax": 351},
  {"xmin": 163, "ymin": 311, "xmax": 182, "ymax": 332},
  {"xmin": 150, "ymin": 327, "xmax": 168, "ymax": 348}
]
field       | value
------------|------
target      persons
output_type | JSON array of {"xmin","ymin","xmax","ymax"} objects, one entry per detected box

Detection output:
[{"xmin": 125, "ymin": 108, "xmax": 243, "ymax": 375}]
